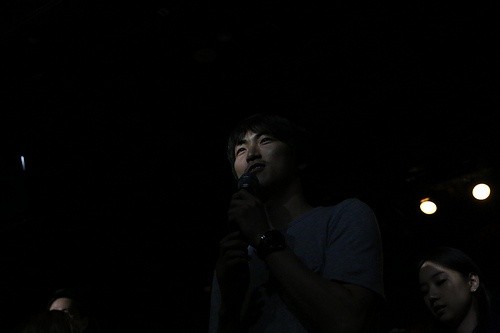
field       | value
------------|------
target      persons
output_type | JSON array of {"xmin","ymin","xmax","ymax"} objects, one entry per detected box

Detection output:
[
  {"xmin": 214, "ymin": 112, "xmax": 394, "ymax": 333},
  {"xmin": 25, "ymin": 296, "xmax": 88, "ymax": 333},
  {"xmin": 417, "ymin": 245, "xmax": 493, "ymax": 333}
]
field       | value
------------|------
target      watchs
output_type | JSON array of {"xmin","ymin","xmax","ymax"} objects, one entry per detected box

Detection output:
[{"xmin": 260, "ymin": 229, "xmax": 287, "ymax": 258}]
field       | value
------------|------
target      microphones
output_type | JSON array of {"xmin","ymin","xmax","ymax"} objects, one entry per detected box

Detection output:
[{"xmin": 236, "ymin": 172, "xmax": 259, "ymax": 246}]
